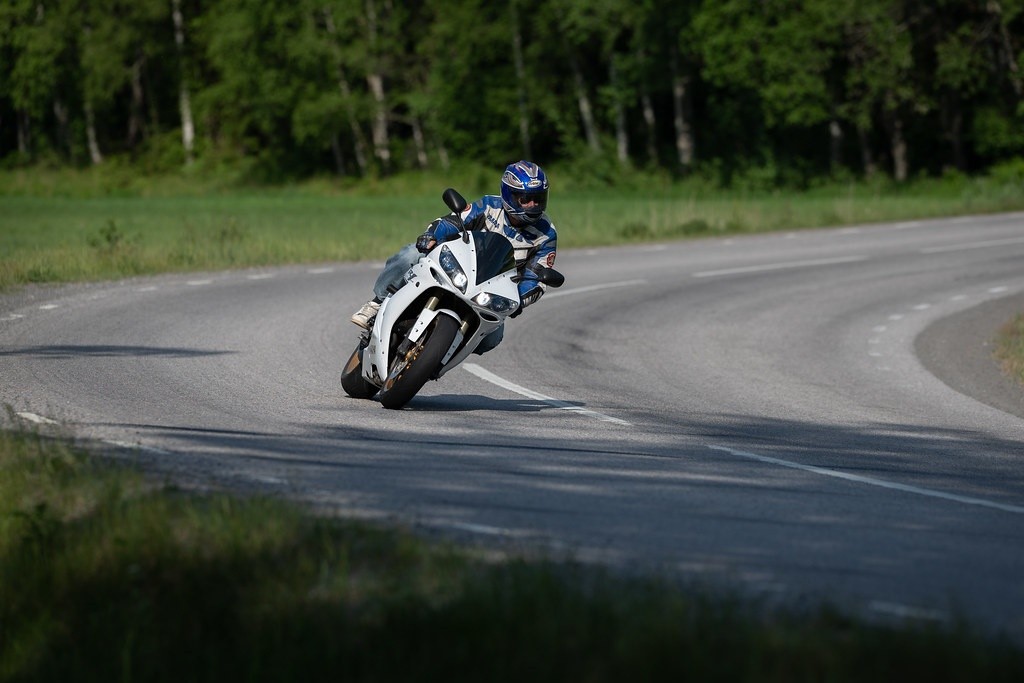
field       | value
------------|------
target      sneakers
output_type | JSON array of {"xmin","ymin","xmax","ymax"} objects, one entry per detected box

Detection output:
[{"xmin": 351, "ymin": 301, "xmax": 379, "ymax": 328}]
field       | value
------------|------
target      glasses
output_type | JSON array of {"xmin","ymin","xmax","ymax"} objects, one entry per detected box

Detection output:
[{"xmin": 520, "ymin": 194, "xmax": 542, "ymax": 204}]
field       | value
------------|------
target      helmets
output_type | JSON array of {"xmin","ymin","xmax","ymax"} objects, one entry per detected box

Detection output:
[{"xmin": 500, "ymin": 160, "xmax": 550, "ymax": 224}]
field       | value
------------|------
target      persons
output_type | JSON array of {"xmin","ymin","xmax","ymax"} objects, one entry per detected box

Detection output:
[{"xmin": 351, "ymin": 160, "xmax": 557, "ymax": 356}]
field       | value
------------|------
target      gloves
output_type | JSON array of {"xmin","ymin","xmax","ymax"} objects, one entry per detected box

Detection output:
[{"xmin": 509, "ymin": 303, "xmax": 522, "ymax": 319}]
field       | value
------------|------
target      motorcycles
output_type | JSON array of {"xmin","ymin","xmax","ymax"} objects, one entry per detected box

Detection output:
[{"xmin": 340, "ymin": 187, "xmax": 565, "ymax": 411}]
